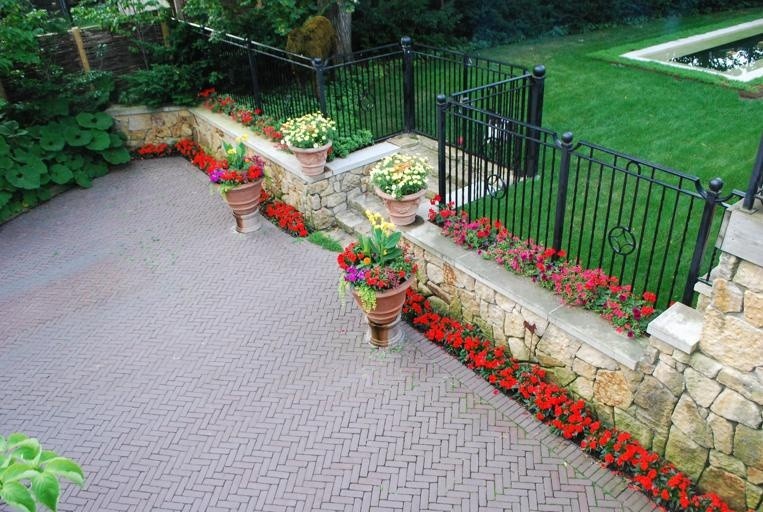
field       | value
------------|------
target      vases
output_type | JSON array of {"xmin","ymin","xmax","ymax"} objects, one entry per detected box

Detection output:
[
  {"xmin": 294, "ymin": 143, "xmax": 330, "ymax": 177},
  {"xmin": 222, "ymin": 183, "xmax": 263, "ymax": 233},
  {"xmin": 342, "ymin": 280, "xmax": 417, "ymax": 348},
  {"xmin": 373, "ymin": 186, "xmax": 426, "ymax": 225}
]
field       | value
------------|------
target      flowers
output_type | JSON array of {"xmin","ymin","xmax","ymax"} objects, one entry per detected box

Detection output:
[
  {"xmin": 201, "ymin": 134, "xmax": 266, "ymax": 183},
  {"xmin": 368, "ymin": 151, "xmax": 432, "ymax": 196},
  {"xmin": 335, "ymin": 208, "xmax": 418, "ymax": 290},
  {"xmin": 282, "ymin": 112, "xmax": 336, "ymax": 148}
]
